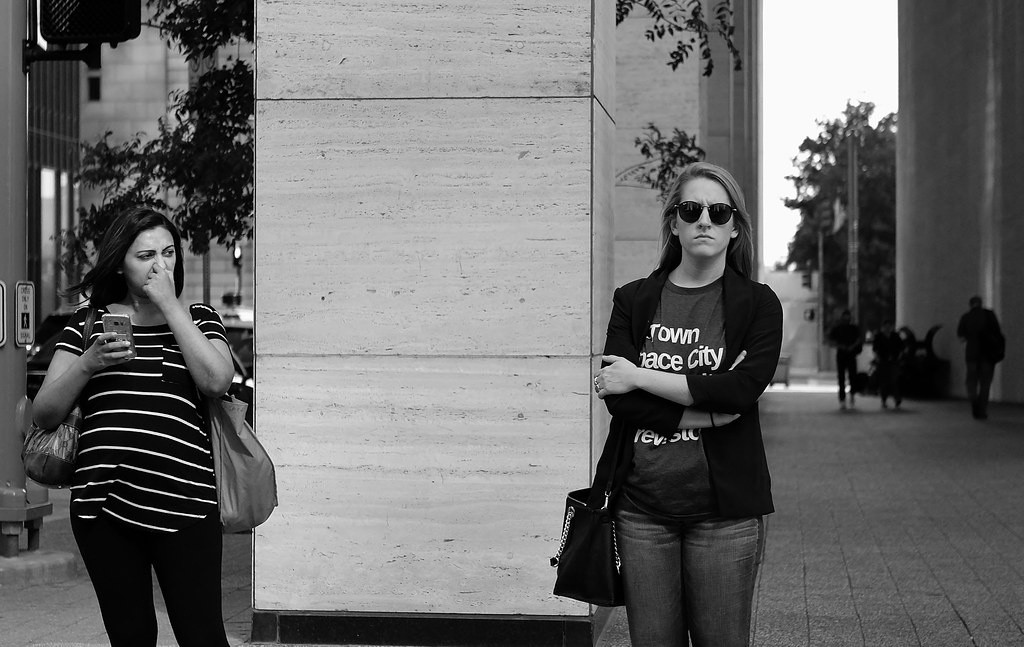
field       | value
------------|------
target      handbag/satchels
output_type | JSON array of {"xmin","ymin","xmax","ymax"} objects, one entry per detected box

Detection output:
[
  {"xmin": 550, "ymin": 489, "xmax": 628, "ymax": 606},
  {"xmin": 21, "ymin": 396, "xmax": 81, "ymax": 486},
  {"xmin": 208, "ymin": 393, "xmax": 278, "ymax": 533}
]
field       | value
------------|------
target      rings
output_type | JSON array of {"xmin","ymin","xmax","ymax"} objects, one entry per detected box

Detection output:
[{"xmin": 594, "ymin": 376, "xmax": 599, "ymax": 389}]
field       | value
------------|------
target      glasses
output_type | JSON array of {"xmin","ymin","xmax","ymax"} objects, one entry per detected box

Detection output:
[{"xmin": 673, "ymin": 201, "xmax": 737, "ymax": 224}]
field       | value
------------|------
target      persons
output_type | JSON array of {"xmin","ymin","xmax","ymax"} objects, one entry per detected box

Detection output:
[
  {"xmin": 828, "ymin": 310, "xmax": 860, "ymax": 411},
  {"xmin": 590, "ymin": 162, "xmax": 783, "ymax": 646},
  {"xmin": 957, "ymin": 297, "xmax": 1005, "ymax": 419},
  {"xmin": 873, "ymin": 319, "xmax": 906, "ymax": 411},
  {"xmin": 30, "ymin": 207, "xmax": 235, "ymax": 647}
]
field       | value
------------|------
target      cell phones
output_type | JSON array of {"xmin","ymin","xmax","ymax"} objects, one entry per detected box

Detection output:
[{"xmin": 101, "ymin": 314, "xmax": 136, "ymax": 359}]
